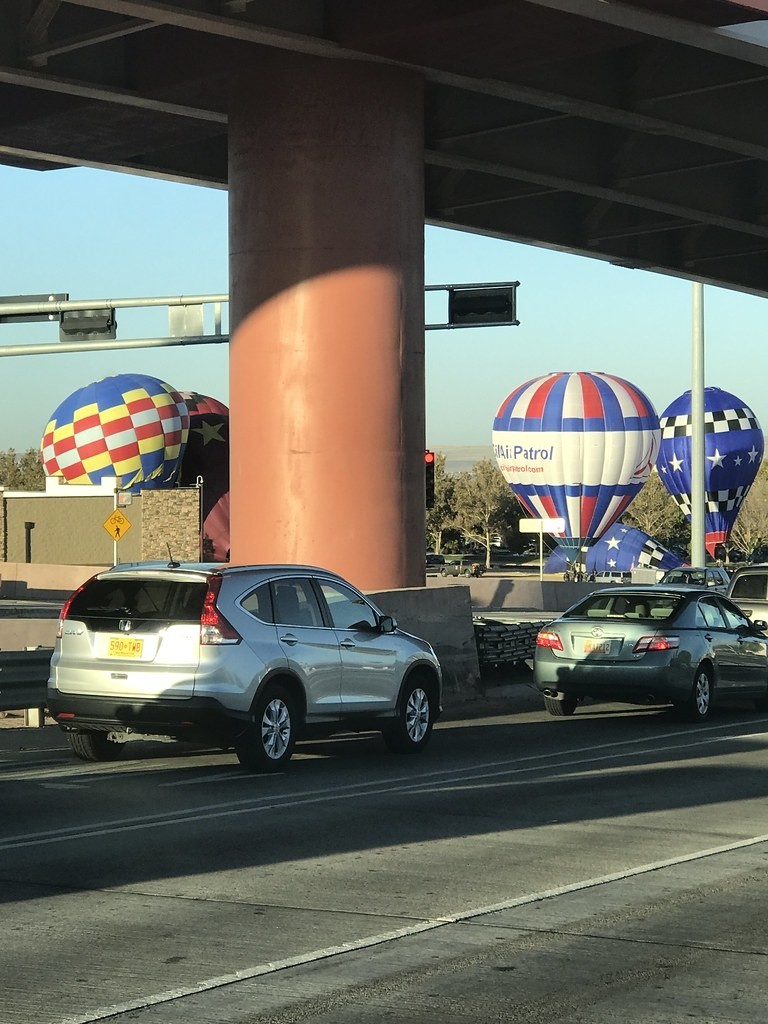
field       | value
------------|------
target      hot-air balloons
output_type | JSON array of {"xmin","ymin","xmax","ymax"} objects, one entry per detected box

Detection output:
[
  {"xmin": 658, "ymin": 386, "xmax": 765, "ymax": 567},
  {"xmin": 541, "ymin": 522, "xmax": 690, "ymax": 578},
  {"xmin": 40, "ymin": 375, "xmax": 233, "ymax": 561},
  {"xmin": 490, "ymin": 371, "xmax": 663, "ymax": 581}
]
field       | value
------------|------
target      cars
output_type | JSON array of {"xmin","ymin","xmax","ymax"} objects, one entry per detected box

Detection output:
[
  {"xmin": 653, "ymin": 567, "xmax": 731, "ymax": 594},
  {"xmin": 533, "ymin": 586, "xmax": 768, "ymax": 722}
]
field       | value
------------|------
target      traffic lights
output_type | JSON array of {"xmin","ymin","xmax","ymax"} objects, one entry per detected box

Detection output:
[{"xmin": 424, "ymin": 451, "xmax": 434, "ymax": 507}]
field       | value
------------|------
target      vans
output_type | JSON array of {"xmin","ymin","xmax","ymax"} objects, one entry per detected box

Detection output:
[{"xmin": 594, "ymin": 571, "xmax": 632, "ymax": 585}]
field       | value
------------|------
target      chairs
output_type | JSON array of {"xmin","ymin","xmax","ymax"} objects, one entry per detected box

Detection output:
[{"xmin": 277, "ymin": 590, "xmax": 314, "ymax": 626}]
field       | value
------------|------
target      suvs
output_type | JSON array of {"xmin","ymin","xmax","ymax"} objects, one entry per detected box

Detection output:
[
  {"xmin": 726, "ymin": 565, "xmax": 768, "ymax": 638},
  {"xmin": 45, "ymin": 560, "xmax": 445, "ymax": 773}
]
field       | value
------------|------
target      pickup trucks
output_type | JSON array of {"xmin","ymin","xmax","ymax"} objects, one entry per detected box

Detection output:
[{"xmin": 442, "ymin": 560, "xmax": 485, "ymax": 579}]
